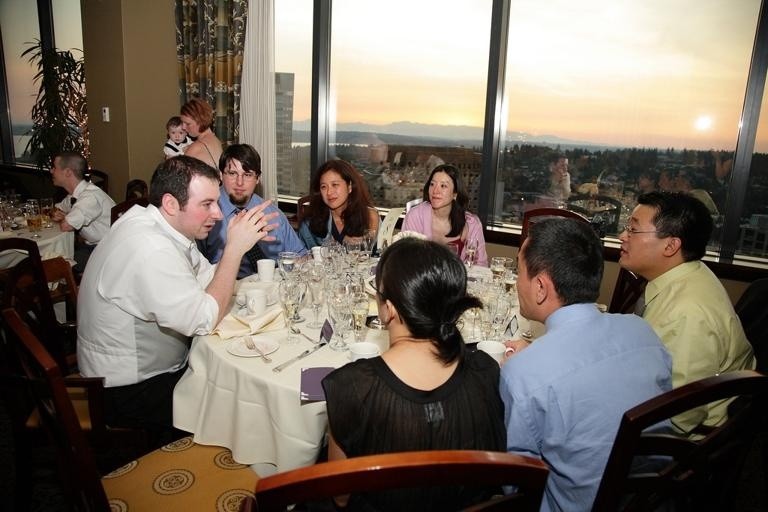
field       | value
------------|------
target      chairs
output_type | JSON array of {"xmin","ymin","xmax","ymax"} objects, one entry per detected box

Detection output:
[
  {"xmin": 583, "ymin": 366, "xmax": 767, "ymax": 510},
  {"xmin": 252, "ymin": 449, "xmax": 555, "ymax": 512},
  {"xmin": 607, "ymin": 249, "xmax": 654, "ymax": 325},
  {"xmin": 0, "ymin": 169, "xmax": 250, "ymax": 510},
  {"xmin": 518, "ymin": 203, "xmax": 598, "ymax": 293},
  {"xmin": 669, "ymin": 270, "xmax": 768, "ymax": 511},
  {"xmin": 293, "ymin": 187, "xmax": 329, "ymax": 238}
]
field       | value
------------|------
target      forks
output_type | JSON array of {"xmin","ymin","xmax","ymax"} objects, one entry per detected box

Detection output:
[{"xmin": 244, "ymin": 331, "xmax": 270, "ymax": 363}]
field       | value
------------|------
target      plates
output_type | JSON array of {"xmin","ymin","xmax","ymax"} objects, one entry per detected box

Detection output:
[
  {"xmin": 235, "ymin": 293, "xmax": 278, "ymax": 306},
  {"xmin": 235, "ymin": 308, "xmax": 267, "ymax": 323},
  {"xmin": 225, "ymin": 335, "xmax": 280, "ymax": 358}
]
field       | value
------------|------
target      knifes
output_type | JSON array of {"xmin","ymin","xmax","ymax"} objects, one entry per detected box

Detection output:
[{"xmin": 273, "ymin": 341, "xmax": 327, "ymax": 372}]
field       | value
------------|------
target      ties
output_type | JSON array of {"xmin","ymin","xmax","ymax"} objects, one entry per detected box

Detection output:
[{"xmin": 236, "ymin": 210, "xmax": 266, "ymax": 273}]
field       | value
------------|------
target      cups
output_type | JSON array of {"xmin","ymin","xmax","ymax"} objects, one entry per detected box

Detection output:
[
  {"xmin": 476, "ymin": 340, "xmax": 516, "ymax": 365},
  {"xmin": 346, "ymin": 342, "xmax": 381, "ymax": 360},
  {"xmin": 256, "ymin": 259, "xmax": 275, "ymax": 284},
  {"xmin": 245, "ymin": 289, "xmax": 267, "ymax": 316}
]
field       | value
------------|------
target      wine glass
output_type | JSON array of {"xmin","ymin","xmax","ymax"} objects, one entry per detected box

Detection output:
[
  {"xmin": 277, "ymin": 240, "xmax": 517, "ymax": 348},
  {"xmin": 0, "ymin": 191, "xmax": 54, "ymax": 239}
]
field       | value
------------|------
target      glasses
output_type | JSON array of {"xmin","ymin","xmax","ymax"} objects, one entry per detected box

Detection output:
[
  {"xmin": 620, "ymin": 221, "xmax": 677, "ymax": 241},
  {"xmin": 222, "ymin": 170, "xmax": 258, "ymax": 183}
]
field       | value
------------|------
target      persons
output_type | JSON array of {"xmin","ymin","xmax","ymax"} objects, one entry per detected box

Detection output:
[
  {"xmin": 319, "ymin": 236, "xmax": 508, "ymax": 506},
  {"xmin": 399, "ymin": 164, "xmax": 489, "ymax": 266},
  {"xmin": 297, "ymin": 159, "xmax": 381, "ymax": 262},
  {"xmin": 615, "ymin": 189, "xmax": 759, "ymax": 445},
  {"xmin": 338, "ymin": 128, "xmax": 447, "ymax": 203},
  {"xmin": 76, "ymin": 153, "xmax": 279, "ymax": 459},
  {"xmin": 163, "ymin": 116, "xmax": 193, "ymax": 158},
  {"xmin": 196, "ymin": 143, "xmax": 309, "ymax": 281},
  {"xmin": 502, "ymin": 152, "xmax": 734, "ymax": 225},
  {"xmin": 180, "ymin": 98, "xmax": 224, "ymax": 175},
  {"xmin": 39, "ymin": 150, "xmax": 123, "ymax": 274},
  {"xmin": 499, "ymin": 216, "xmax": 674, "ymax": 510}
]
